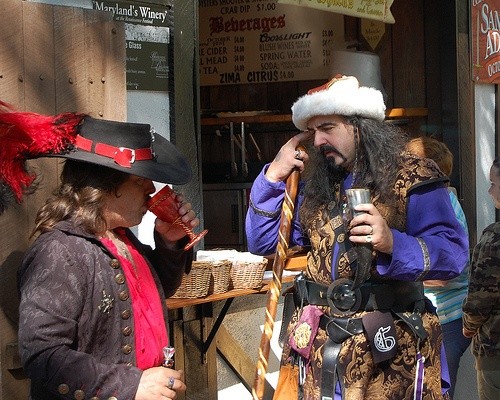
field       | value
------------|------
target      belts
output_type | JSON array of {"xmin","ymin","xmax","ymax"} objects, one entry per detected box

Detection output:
[{"xmin": 304, "ymin": 280, "xmax": 422, "ymax": 312}]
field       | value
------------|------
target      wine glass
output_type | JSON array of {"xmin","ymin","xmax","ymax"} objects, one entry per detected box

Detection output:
[{"xmin": 145, "ymin": 185, "xmax": 208, "ymax": 250}]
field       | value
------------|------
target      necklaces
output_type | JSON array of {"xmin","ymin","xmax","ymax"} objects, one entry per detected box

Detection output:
[{"xmin": 122, "ymin": 246, "xmax": 129, "ymax": 259}]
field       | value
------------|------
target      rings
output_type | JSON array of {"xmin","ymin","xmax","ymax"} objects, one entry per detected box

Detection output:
[
  {"xmin": 296, "ymin": 151, "xmax": 300, "ymax": 158},
  {"xmin": 370, "ymin": 226, "xmax": 373, "ymax": 234},
  {"xmin": 366, "ymin": 235, "xmax": 372, "ymax": 243},
  {"xmin": 169, "ymin": 377, "xmax": 174, "ymax": 388}
]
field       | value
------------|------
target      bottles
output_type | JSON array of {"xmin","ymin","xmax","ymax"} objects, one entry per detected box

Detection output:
[{"xmin": 163, "ymin": 347, "xmax": 176, "ymax": 370}]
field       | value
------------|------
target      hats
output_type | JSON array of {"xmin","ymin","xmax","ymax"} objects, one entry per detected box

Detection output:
[
  {"xmin": 292, "ymin": 74, "xmax": 385, "ymax": 130},
  {"xmin": 0, "ymin": 102, "xmax": 192, "ymax": 215}
]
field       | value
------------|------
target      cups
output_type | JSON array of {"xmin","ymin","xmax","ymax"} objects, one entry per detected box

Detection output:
[{"xmin": 345, "ymin": 189, "xmax": 371, "ymax": 227}]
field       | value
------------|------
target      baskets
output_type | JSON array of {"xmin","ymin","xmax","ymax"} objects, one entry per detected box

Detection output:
[
  {"xmin": 232, "ymin": 258, "xmax": 269, "ymax": 289},
  {"xmin": 209, "ymin": 261, "xmax": 233, "ymax": 293},
  {"xmin": 169, "ymin": 262, "xmax": 212, "ymax": 299}
]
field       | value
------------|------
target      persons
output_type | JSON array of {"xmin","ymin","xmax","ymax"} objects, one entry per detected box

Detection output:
[
  {"xmin": 460, "ymin": 155, "xmax": 500, "ymax": 400},
  {"xmin": 245, "ymin": 76, "xmax": 470, "ymax": 400},
  {"xmin": 405, "ymin": 136, "xmax": 470, "ymax": 399},
  {"xmin": 15, "ymin": 118, "xmax": 199, "ymax": 400}
]
